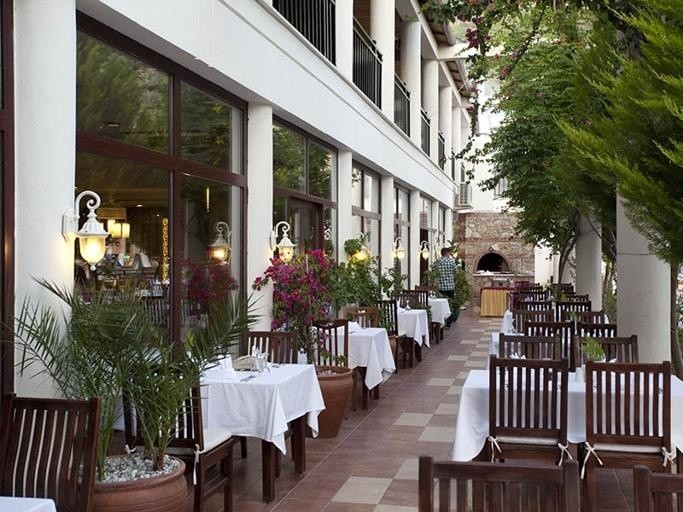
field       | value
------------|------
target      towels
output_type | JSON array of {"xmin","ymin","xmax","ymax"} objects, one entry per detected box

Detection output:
[
  {"xmin": 218, "ymin": 355, "xmax": 235, "ymax": 379},
  {"xmin": 511, "ymin": 352, "xmax": 535, "ymax": 382},
  {"xmin": 348, "ymin": 319, "xmax": 361, "ymax": 334}
]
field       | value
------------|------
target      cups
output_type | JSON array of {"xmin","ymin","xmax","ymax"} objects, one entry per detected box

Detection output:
[
  {"xmin": 575, "ymin": 368, "xmax": 584, "ymax": 384},
  {"xmin": 580, "ymin": 364, "xmax": 588, "ymax": 383},
  {"xmin": 218, "ymin": 355, "xmax": 232, "ymax": 373}
]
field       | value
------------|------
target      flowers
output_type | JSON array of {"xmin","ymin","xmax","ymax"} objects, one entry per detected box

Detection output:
[{"xmin": 250, "ymin": 249, "xmax": 349, "ymax": 374}]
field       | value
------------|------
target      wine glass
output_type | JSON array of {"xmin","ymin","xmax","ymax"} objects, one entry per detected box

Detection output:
[{"xmin": 259, "ymin": 354, "xmax": 271, "ymax": 378}]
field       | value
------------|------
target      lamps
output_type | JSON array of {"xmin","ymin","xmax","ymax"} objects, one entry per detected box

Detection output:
[
  {"xmin": 270, "ymin": 222, "xmax": 296, "ymax": 267},
  {"xmin": 420, "ymin": 240, "xmax": 430, "ymax": 261},
  {"xmin": 393, "ymin": 235, "xmax": 405, "ymax": 260},
  {"xmin": 61, "ymin": 191, "xmax": 110, "ymax": 271},
  {"xmin": 208, "ymin": 221, "xmax": 232, "ymax": 264},
  {"xmin": 347, "ymin": 233, "xmax": 369, "ymax": 266}
]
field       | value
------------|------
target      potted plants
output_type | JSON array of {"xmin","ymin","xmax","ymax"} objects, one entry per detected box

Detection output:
[
  {"xmin": 375, "ymin": 259, "xmax": 410, "ymax": 308},
  {"xmin": 1, "ymin": 270, "xmax": 266, "ymax": 511},
  {"xmin": 329, "ymin": 258, "xmax": 398, "ymax": 377},
  {"xmin": 423, "ymin": 241, "xmax": 471, "ymax": 322}
]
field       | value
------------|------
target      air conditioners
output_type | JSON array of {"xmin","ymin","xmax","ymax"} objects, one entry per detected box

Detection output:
[{"xmin": 456, "ymin": 182, "xmax": 473, "ymax": 210}]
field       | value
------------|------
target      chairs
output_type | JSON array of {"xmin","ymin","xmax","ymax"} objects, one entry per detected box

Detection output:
[
  {"xmin": 417, "ymin": 455, "xmax": 580, "ymax": 512},
  {"xmin": 575, "ymin": 321, "xmax": 617, "ymax": 338},
  {"xmin": 556, "ymin": 298, "xmax": 592, "ymax": 322},
  {"xmin": 344, "ymin": 307, "xmax": 381, "ymax": 399},
  {"xmin": 560, "ymin": 308, "xmax": 605, "ymax": 323},
  {"xmin": 1, "ymin": 391, "xmax": 101, "ymax": 511},
  {"xmin": 487, "ymin": 358, "xmax": 568, "ymax": 512},
  {"xmin": 574, "ymin": 333, "xmax": 640, "ymax": 372},
  {"xmin": 511, "ymin": 309, "xmax": 554, "ymax": 334},
  {"xmin": 498, "ymin": 331, "xmax": 562, "ymax": 370},
  {"xmin": 403, "ymin": 289, "xmax": 431, "ymax": 347},
  {"xmin": 316, "ymin": 320, "xmax": 349, "ymax": 371},
  {"xmin": 583, "ymin": 359, "xmax": 672, "ymax": 512},
  {"xmin": 498, "ymin": 335, "xmax": 562, "ymax": 362},
  {"xmin": 524, "ymin": 319, "xmax": 571, "ymax": 360},
  {"xmin": 377, "ymin": 300, "xmax": 400, "ymax": 374},
  {"xmin": 633, "ymin": 463, "xmax": 683, "ymax": 512},
  {"xmin": 239, "ymin": 328, "xmax": 297, "ymax": 478},
  {"xmin": 517, "ymin": 299, "xmax": 553, "ymax": 310},
  {"xmin": 121, "ymin": 361, "xmax": 241, "ymax": 512}
]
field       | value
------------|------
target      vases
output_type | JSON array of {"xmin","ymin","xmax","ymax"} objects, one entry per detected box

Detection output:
[{"xmin": 300, "ymin": 362, "xmax": 353, "ymax": 440}]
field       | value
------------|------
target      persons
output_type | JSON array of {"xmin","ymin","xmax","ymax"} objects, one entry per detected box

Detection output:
[{"xmin": 431, "ymin": 247, "xmax": 457, "ymax": 329}]
